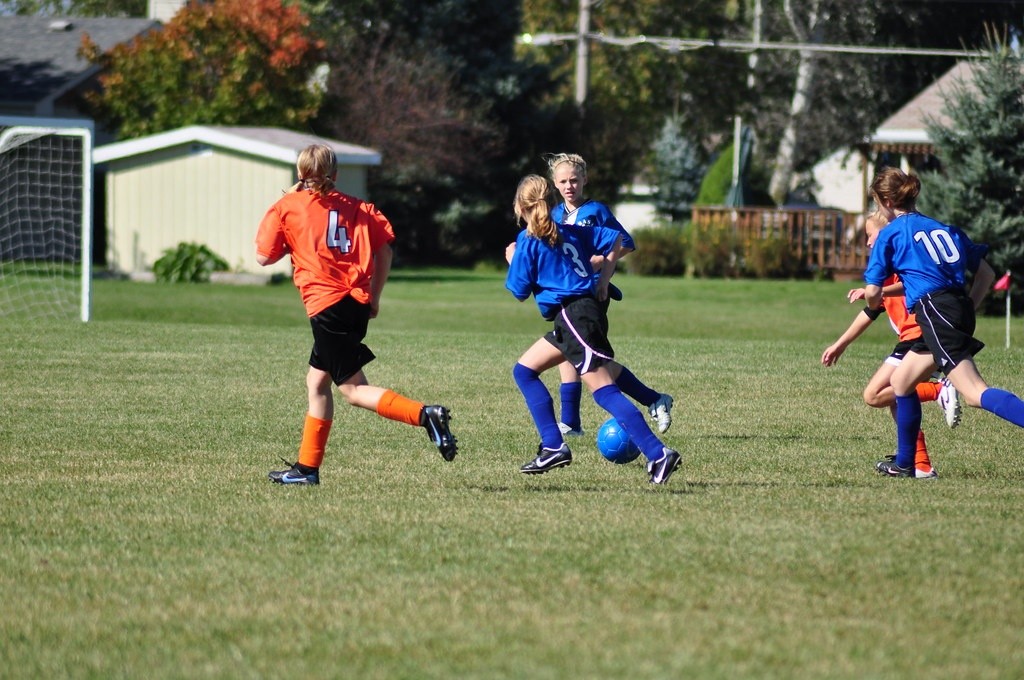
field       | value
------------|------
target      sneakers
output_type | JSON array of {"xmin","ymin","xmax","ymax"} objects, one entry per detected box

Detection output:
[
  {"xmin": 648, "ymin": 393, "xmax": 673, "ymax": 433},
  {"xmin": 268, "ymin": 458, "xmax": 319, "ymax": 485},
  {"xmin": 520, "ymin": 443, "xmax": 572, "ymax": 475},
  {"xmin": 936, "ymin": 377, "xmax": 962, "ymax": 429},
  {"xmin": 915, "ymin": 467, "xmax": 938, "ymax": 478},
  {"xmin": 557, "ymin": 423, "xmax": 585, "ymax": 436},
  {"xmin": 646, "ymin": 447, "xmax": 682, "ymax": 485},
  {"xmin": 875, "ymin": 455, "xmax": 916, "ymax": 478},
  {"xmin": 419, "ymin": 405, "xmax": 459, "ymax": 462}
]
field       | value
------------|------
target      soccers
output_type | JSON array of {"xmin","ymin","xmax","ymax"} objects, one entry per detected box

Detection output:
[{"xmin": 597, "ymin": 417, "xmax": 641, "ymax": 464}]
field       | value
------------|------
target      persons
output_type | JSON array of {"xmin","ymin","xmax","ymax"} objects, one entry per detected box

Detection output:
[
  {"xmin": 821, "ymin": 169, "xmax": 1024, "ymax": 479},
  {"xmin": 505, "ymin": 153, "xmax": 683, "ymax": 485},
  {"xmin": 255, "ymin": 143, "xmax": 458, "ymax": 484}
]
